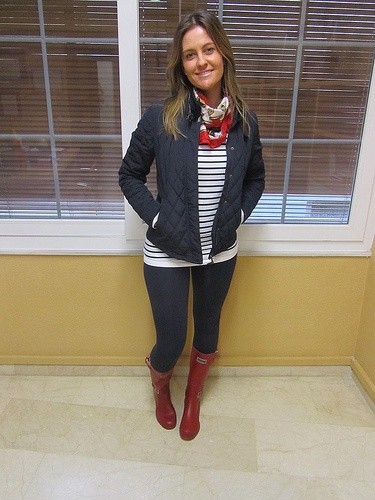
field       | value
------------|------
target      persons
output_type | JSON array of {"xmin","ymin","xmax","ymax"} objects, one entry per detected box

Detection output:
[{"xmin": 118, "ymin": 10, "xmax": 265, "ymax": 441}]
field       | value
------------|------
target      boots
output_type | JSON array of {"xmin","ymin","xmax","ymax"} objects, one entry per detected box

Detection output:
[
  {"xmin": 179, "ymin": 343, "xmax": 218, "ymax": 440},
  {"xmin": 145, "ymin": 353, "xmax": 177, "ymax": 430}
]
